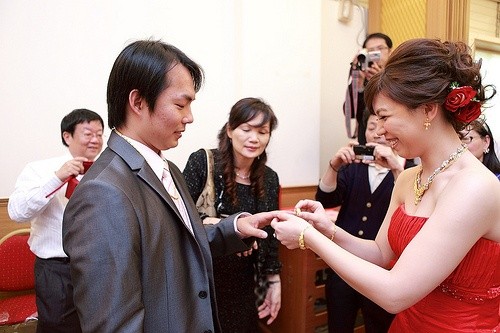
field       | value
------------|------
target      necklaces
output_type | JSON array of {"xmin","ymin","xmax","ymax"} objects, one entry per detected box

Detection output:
[
  {"xmin": 415, "ymin": 142, "xmax": 467, "ymax": 205},
  {"xmin": 233, "ymin": 170, "xmax": 251, "ymax": 179}
]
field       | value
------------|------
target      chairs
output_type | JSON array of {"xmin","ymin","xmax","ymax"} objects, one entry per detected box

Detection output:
[{"xmin": 0, "ymin": 227, "xmax": 43, "ymax": 333}]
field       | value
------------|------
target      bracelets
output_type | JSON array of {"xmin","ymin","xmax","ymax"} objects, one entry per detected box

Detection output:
[
  {"xmin": 299, "ymin": 224, "xmax": 314, "ymax": 250},
  {"xmin": 329, "ymin": 223, "xmax": 336, "ymax": 241},
  {"xmin": 263, "ymin": 280, "xmax": 281, "ymax": 289}
]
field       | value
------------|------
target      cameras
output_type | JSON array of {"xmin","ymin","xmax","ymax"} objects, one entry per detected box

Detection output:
[
  {"xmin": 79, "ymin": 161, "xmax": 94, "ymax": 175},
  {"xmin": 357, "ymin": 48, "xmax": 382, "ymax": 71},
  {"xmin": 354, "ymin": 145, "xmax": 375, "ymax": 160}
]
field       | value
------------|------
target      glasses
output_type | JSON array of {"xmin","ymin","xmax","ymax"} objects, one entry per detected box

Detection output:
[
  {"xmin": 461, "ymin": 134, "xmax": 486, "ymax": 143},
  {"xmin": 367, "ymin": 45, "xmax": 390, "ymax": 53},
  {"xmin": 71, "ymin": 131, "xmax": 107, "ymax": 140}
]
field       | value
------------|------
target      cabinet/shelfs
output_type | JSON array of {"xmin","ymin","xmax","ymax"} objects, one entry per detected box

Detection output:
[{"xmin": 272, "ymin": 207, "xmax": 368, "ymax": 333}]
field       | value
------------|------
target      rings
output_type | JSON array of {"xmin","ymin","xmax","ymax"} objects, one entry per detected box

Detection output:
[{"xmin": 295, "ymin": 208, "xmax": 302, "ymax": 218}]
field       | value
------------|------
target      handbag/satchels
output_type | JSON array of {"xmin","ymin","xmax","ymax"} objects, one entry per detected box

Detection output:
[{"xmin": 194, "ymin": 148, "xmax": 217, "ymax": 218}]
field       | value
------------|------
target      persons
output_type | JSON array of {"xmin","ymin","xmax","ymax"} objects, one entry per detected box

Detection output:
[
  {"xmin": 270, "ymin": 38, "xmax": 500, "ymax": 333},
  {"xmin": 184, "ymin": 98, "xmax": 281, "ymax": 333},
  {"xmin": 456, "ymin": 119, "xmax": 500, "ymax": 181},
  {"xmin": 7, "ymin": 109, "xmax": 104, "ymax": 333},
  {"xmin": 63, "ymin": 40, "xmax": 300, "ymax": 333},
  {"xmin": 343, "ymin": 33, "xmax": 415, "ymax": 170},
  {"xmin": 315, "ymin": 105, "xmax": 419, "ymax": 333}
]
